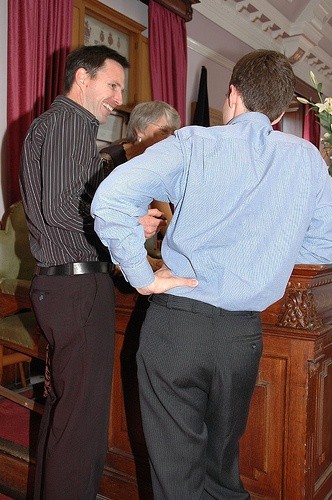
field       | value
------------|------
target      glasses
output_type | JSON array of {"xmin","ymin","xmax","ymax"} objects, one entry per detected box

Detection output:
[{"xmin": 149, "ymin": 122, "xmax": 177, "ymax": 135}]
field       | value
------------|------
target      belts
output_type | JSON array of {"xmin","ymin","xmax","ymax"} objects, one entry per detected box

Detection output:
[
  {"xmin": 151, "ymin": 292, "xmax": 256, "ymax": 316},
  {"xmin": 36, "ymin": 260, "xmax": 116, "ymax": 275}
]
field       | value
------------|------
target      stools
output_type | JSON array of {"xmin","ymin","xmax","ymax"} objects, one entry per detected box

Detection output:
[{"xmin": 0, "ymin": 312, "xmax": 50, "ymax": 415}]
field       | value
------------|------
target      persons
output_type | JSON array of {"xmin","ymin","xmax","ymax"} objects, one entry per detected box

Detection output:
[
  {"xmin": 91, "ymin": 49, "xmax": 332, "ymax": 499},
  {"xmin": 100, "ymin": 100, "xmax": 173, "ymax": 238},
  {"xmin": 20, "ymin": 44, "xmax": 130, "ymax": 499}
]
